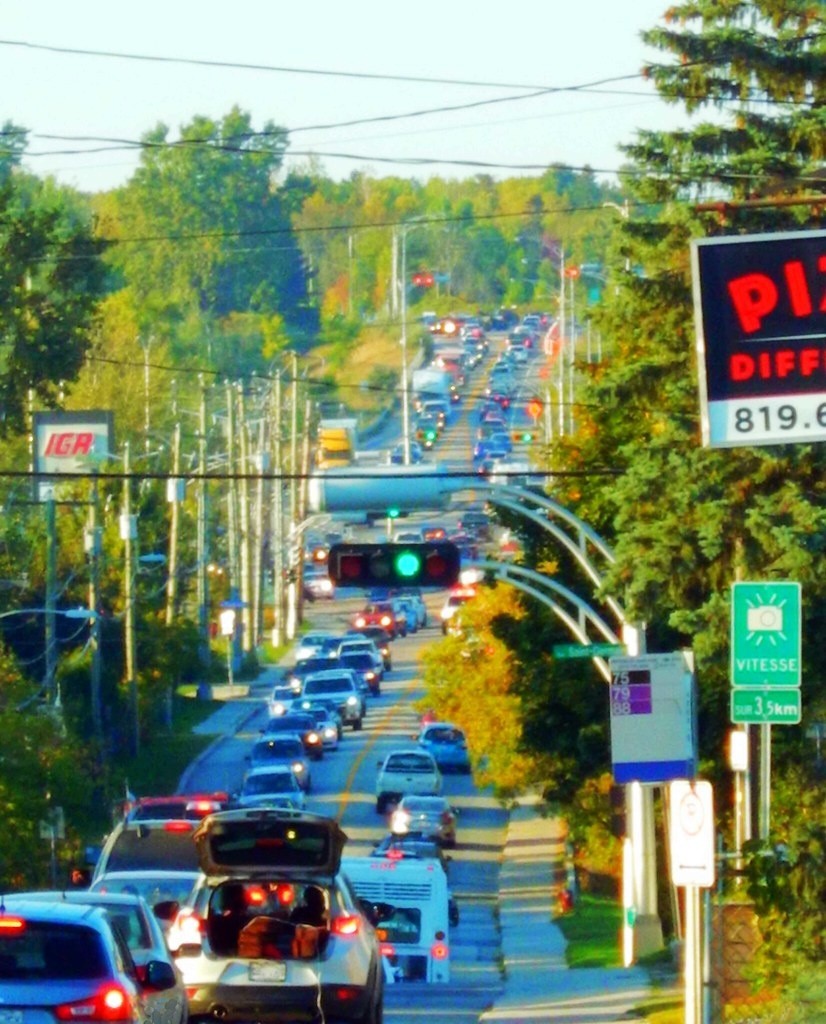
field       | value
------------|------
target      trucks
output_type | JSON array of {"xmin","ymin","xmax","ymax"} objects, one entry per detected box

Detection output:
[{"xmin": 314, "ymin": 417, "xmax": 358, "ymax": 470}]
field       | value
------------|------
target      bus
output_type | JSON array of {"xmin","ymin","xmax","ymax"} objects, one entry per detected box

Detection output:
[{"xmin": 338, "ymin": 851, "xmax": 460, "ymax": 991}]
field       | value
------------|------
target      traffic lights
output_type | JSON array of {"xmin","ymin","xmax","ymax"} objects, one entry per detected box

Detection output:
[{"xmin": 326, "ymin": 544, "xmax": 461, "ymax": 589}]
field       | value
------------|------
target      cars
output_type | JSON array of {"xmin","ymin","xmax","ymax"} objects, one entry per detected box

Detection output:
[
  {"xmin": 1, "ymin": 900, "xmax": 178, "ymax": 1024},
  {"xmin": 132, "ymin": 794, "xmax": 228, "ymax": 820},
  {"xmin": 0, "ymin": 892, "xmax": 191, "ymax": 1024},
  {"xmin": 392, "ymin": 795, "xmax": 461, "ymax": 849},
  {"xmin": 243, "ymin": 735, "xmax": 313, "ymax": 793},
  {"xmin": 389, "ymin": 304, "xmax": 551, "ymax": 480},
  {"xmin": 236, "ymin": 767, "xmax": 311, "ymax": 816},
  {"xmin": 86, "ymin": 818, "xmax": 207, "ymax": 938},
  {"xmin": 409, "ymin": 723, "xmax": 472, "ymax": 776},
  {"xmin": 261, "ymin": 512, "xmax": 493, "ymax": 751},
  {"xmin": 377, "ymin": 748, "xmax": 443, "ymax": 816},
  {"xmin": 372, "ymin": 831, "xmax": 452, "ymax": 874}
]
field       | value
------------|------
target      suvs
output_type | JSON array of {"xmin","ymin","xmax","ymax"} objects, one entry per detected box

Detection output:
[{"xmin": 152, "ymin": 808, "xmax": 397, "ymax": 1024}]
